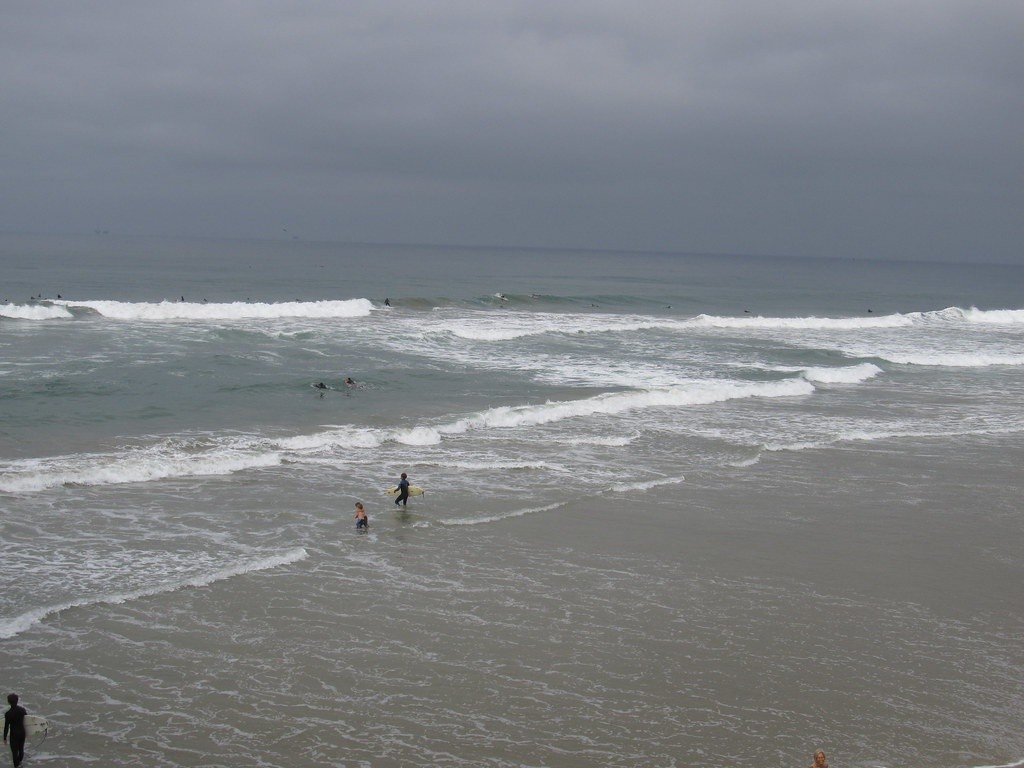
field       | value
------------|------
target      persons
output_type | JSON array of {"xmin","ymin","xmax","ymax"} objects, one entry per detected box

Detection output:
[
  {"xmin": 347, "ymin": 378, "xmax": 354, "ymax": 384},
  {"xmin": 384, "ymin": 298, "xmax": 389, "ymax": 306},
  {"xmin": 181, "ymin": 296, "xmax": 184, "ymax": 301},
  {"xmin": 317, "ymin": 383, "xmax": 325, "ymax": 388},
  {"xmin": 394, "ymin": 473, "xmax": 410, "ymax": 506},
  {"xmin": 3, "ymin": 693, "xmax": 27, "ymax": 768},
  {"xmin": 354, "ymin": 502, "xmax": 367, "ymax": 528}
]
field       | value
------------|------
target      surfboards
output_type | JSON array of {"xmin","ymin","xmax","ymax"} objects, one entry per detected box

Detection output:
[{"xmin": 386, "ymin": 486, "xmax": 426, "ymax": 496}]
